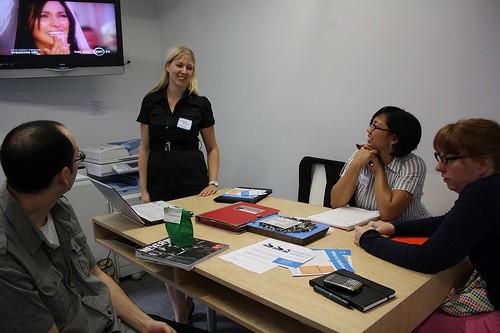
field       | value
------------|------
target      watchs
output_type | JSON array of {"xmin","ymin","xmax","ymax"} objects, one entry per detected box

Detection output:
[{"xmin": 208, "ymin": 181, "xmax": 219, "ymax": 186}]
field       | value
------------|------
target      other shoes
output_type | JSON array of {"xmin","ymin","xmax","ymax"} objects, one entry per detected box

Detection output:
[{"xmin": 174, "ymin": 301, "xmax": 195, "ymax": 325}]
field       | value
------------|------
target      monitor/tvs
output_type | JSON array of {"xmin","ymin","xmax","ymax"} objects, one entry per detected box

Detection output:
[{"xmin": 0, "ymin": 0, "xmax": 124, "ymax": 78}]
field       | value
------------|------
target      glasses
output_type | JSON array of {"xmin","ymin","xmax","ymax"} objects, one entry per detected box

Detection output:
[
  {"xmin": 434, "ymin": 152, "xmax": 483, "ymax": 168},
  {"xmin": 370, "ymin": 122, "xmax": 390, "ymax": 131},
  {"xmin": 57, "ymin": 151, "xmax": 86, "ymax": 173}
]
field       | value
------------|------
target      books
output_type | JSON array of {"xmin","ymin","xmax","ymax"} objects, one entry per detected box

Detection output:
[
  {"xmin": 196, "ymin": 200, "xmax": 280, "ymax": 232},
  {"xmin": 307, "ymin": 269, "xmax": 398, "ymax": 313},
  {"xmin": 212, "ymin": 185, "xmax": 272, "ymax": 205},
  {"xmin": 309, "ymin": 203, "xmax": 382, "ymax": 230},
  {"xmin": 135, "ymin": 236, "xmax": 230, "ymax": 271}
]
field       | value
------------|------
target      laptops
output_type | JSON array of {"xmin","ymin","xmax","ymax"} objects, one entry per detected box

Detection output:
[{"xmin": 88, "ymin": 177, "xmax": 194, "ymax": 226}]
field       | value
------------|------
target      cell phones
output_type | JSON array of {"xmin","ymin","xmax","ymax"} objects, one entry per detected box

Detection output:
[{"xmin": 324, "ymin": 273, "xmax": 362, "ymax": 292}]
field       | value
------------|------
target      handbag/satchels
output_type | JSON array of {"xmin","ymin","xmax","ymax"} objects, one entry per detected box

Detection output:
[{"xmin": 441, "ymin": 269, "xmax": 494, "ymax": 317}]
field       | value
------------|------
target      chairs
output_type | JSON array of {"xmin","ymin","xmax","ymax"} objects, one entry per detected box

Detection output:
[
  {"xmin": 420, "ymin": 171, "xmax": 460, "ymax": 217},
  {"xmin": 297, "ymin": 155, "xmax": 358, "ymax": 209}
]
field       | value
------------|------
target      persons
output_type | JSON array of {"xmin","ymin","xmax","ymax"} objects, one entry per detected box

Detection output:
[
  {"xmin": 136, "ymin": 44, "xmax": 220, "ymax": 330},
  {"xmin": 76, "ymin": 24, "xmax": 103, "ymax": 52},
  {"xmin": 0, "ymin": 118, "xmax": 212, "ymax": 333},
  {"xmin": 331, "ymin": 106, "xmax": 434, "ymax": 230},
  {"xmin": 101, "ymin": 23, "xmax": 119, "ymax": 53},
  {"xmin": 9, "ymin": 0, "xmax": 83, "ymax": 56},
  {"xmin": 354, "ymin": 118, "xmax": 500, "ymax": 333}
]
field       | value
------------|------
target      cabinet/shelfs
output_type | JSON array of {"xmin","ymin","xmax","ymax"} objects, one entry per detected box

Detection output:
[{"xmin": 61, "ymin": 166, "xmax": 145, "ymax": 279}]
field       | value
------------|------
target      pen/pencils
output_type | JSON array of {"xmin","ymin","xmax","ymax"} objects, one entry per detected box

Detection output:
[
  {"xmin": 313, "ymin": 285, "xmax": 349, "ymax": 306},
  {"xmin": 355, "ymin": 143, "xmax": 374, "ymax": 167}
]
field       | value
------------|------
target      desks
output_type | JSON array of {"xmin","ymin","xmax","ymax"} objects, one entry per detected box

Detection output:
[{"xmin": 91, "ymin": 187, "xmax": 472, "ymax": 333}]
